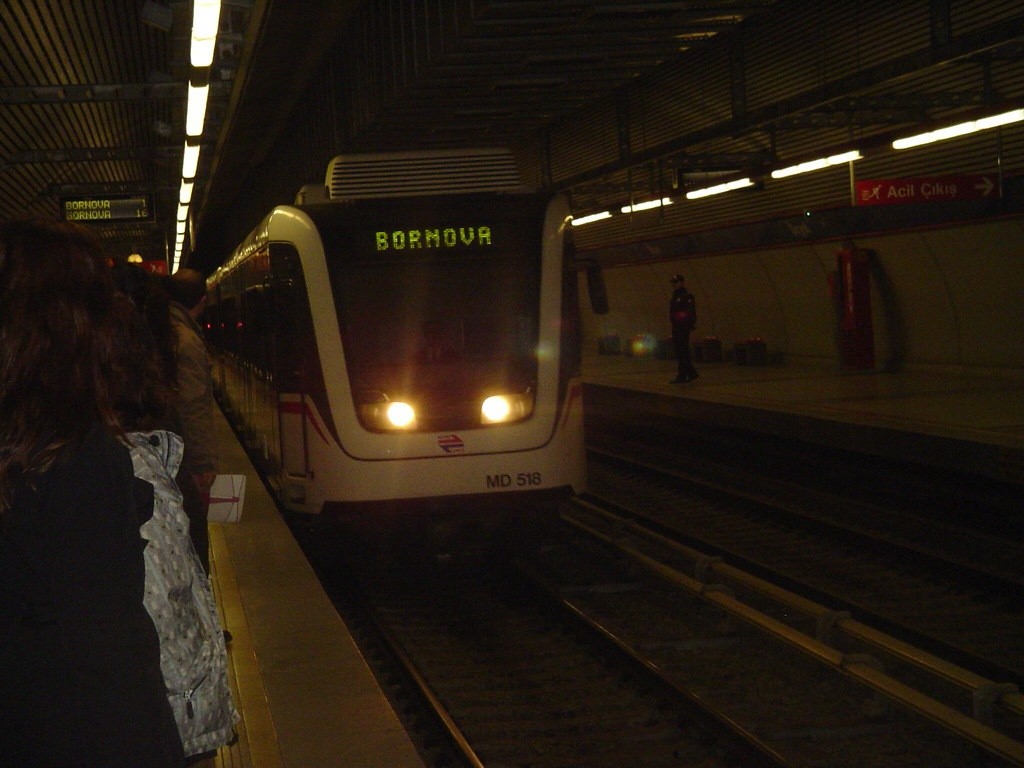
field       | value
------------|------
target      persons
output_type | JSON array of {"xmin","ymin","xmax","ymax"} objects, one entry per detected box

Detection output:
[
  {"xmin": 94, "ymin": 284, "xmax": 241, "ymax": 761},
  {"xmin": 668, "ymin": 274, "xmax": 700, "ymax": 385},
  {"xmin": 164, "ymin": 264, "xmax": 234, "ymax": 646},
  {"xmin": 1, "ymin": 219, "xmax": 199, "ymax": 767}
]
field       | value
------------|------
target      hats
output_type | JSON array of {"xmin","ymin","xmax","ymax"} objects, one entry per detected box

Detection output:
[{"xmin": 670, "ymin": 275, "xmax": 684, "ymax": 282}]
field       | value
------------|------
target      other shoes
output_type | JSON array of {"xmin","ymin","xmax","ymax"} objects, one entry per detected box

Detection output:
[
  {"xmin": 669, "ymin": 376, "xmax": 689, "ymax": 384},
  {"xmin": 687, "ymin": 374, "xmax": 699, "ymax": 382}
]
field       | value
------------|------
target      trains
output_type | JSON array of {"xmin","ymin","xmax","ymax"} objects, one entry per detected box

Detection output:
[{"xmin": 191, "ymin": 146, "xmax": 608, "ymax": 542}]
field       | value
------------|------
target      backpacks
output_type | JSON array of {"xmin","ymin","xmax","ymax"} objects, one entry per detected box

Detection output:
[{"xmin": 1, "ymin": 431, "xmax": 186, "ymax": 766}]
